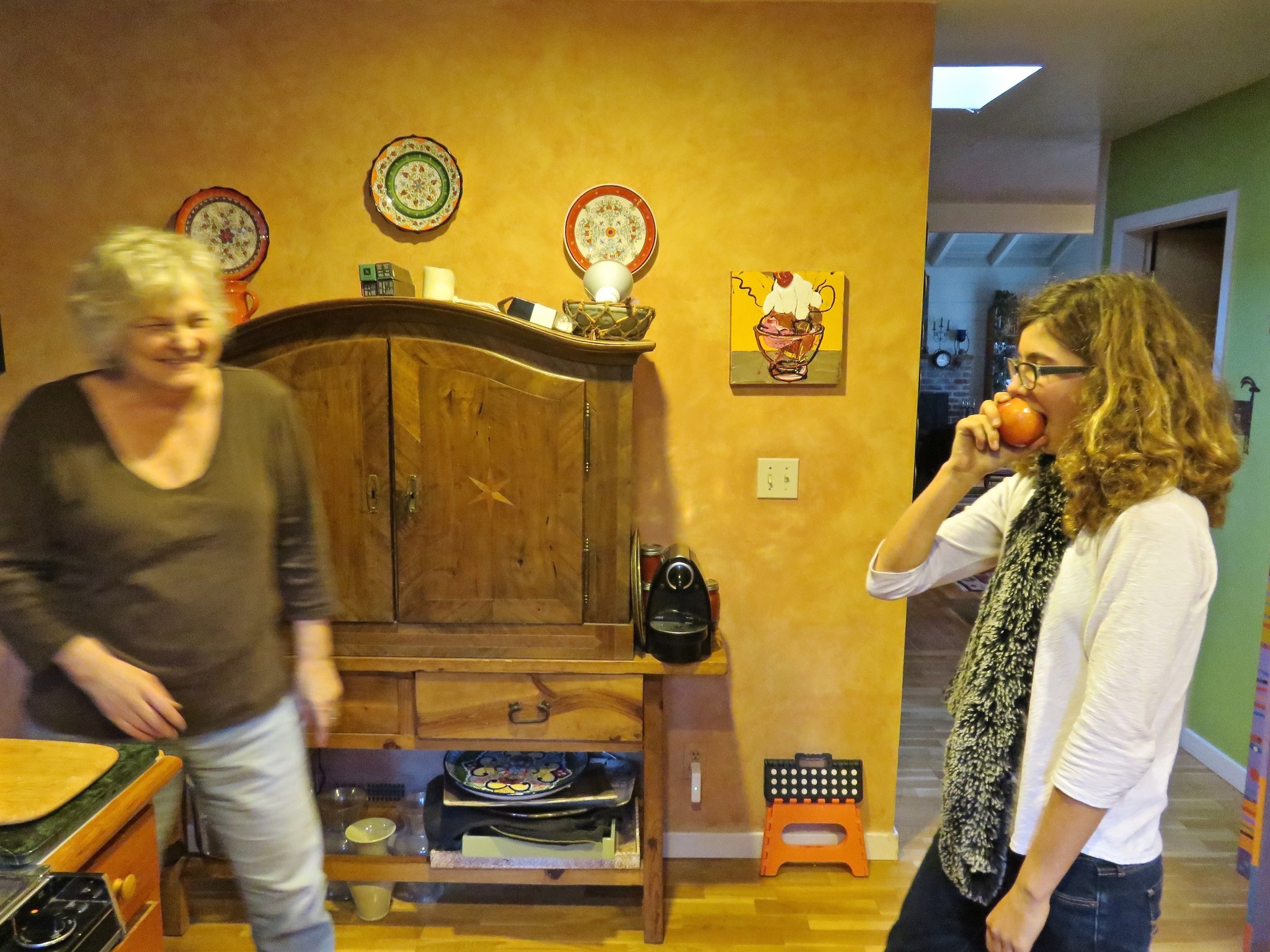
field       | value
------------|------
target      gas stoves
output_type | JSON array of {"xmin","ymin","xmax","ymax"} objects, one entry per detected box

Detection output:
[{"xmin": 0, "ymin": 864, "xmax": 130, "ymax": 951}]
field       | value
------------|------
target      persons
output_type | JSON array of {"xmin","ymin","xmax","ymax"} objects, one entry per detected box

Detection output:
[
  {"xmin": 0, "ymin": 223, "xmax": 346, "ymax": 952},
  {"xmin": 867, "ymin": 268, "xmax": 1245, "ymax": 949}
]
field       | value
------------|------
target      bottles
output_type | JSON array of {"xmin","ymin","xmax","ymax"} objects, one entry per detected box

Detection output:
[{"xmin": 317, "ymin": 786, "xmax": 445, "ymax": 920}]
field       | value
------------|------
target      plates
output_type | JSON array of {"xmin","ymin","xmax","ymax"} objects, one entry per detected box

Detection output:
[
  {"xmin": 175, "ymin": 187, "xmax": 269, "ymax": 280},
  {"xmin": 443, "ymin": 750, "xmax": 635, "ymax": 819},
  {"xmin": 564, "ymin": 183, "xmax": 657, "ymax": 274},
  {"xmin": 369, "ymin": 133, "xmax": 464, "ymax": 234}
]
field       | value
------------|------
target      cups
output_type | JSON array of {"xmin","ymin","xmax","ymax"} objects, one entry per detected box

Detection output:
[
  {"xmin": 640, "ymin": 543, "xmax": 664, "ymax": 610},
  {"xmin": 225, "ymin": 280, "xmax": 259, "ymax": 328},
  {"xmin": 704, "ymin": 579, "xmax": 720, "ymax": 632}
]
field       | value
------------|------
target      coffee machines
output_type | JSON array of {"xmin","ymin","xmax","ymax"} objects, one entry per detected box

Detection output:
[{"xmin": 646, "ymin": 543, "xmax": 713, "ymax": 662}]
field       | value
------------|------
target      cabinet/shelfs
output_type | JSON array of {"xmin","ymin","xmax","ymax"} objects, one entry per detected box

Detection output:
[{"xmin": 186, "ymin": 288, "xmax": 659, "ymax": 660}]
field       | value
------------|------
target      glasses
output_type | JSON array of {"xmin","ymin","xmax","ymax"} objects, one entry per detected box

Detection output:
[{"xmin": 1007, "ymin": 358, "xmax": 1095, "ymax": 391}]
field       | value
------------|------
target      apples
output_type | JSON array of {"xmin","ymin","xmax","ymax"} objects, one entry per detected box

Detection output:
[{"xmin": 994, "ymin": 397, "xmax": 1045, "ymax": 448}]
